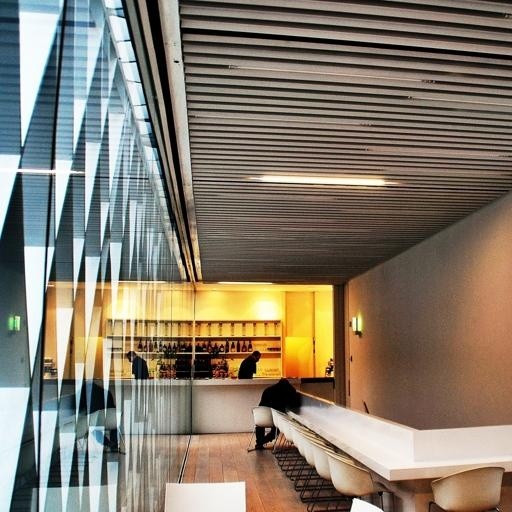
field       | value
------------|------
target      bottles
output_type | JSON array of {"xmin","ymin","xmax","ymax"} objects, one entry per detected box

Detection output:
[
  {"xmin": 196, "ymin": 337, "xmax": 253, "ymax": 353},
  {"xmin": 135, "ymin": 339, "xmax": 192, "ymax": 355}
]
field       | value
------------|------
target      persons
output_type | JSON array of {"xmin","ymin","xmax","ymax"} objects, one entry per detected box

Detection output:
[
  {"xmin": 237, "ymin": 351, "xmax": 261, "ymax": 379},
  {"xmin": 125, "ymin": 350, "xmax": 149, "ymax": 379},
  {"xmin": 72, "ymin": 381, "xmax": 118, "ymax": 453},
  {"xmin": 255, "ymin": 376, "xmax": 297, "ymax": 448}
]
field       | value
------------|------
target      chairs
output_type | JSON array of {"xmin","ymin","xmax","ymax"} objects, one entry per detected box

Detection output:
[
  {"xmin": 164, "ymin": 480, "xmax": 248, "ymax": 512},
  {"xmin": 425, "ymin": 465, "xmax": 506, "ymax": 512},
  {"xmin": 270, "ymin": 397, "xmax": 395, "ymax": 510},
  {"xmin": 246, "ymin": 407, "xmax": 274, "ymax": 452}
]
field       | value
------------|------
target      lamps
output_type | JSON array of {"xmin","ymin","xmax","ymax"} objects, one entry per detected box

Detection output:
[{"xmin": 345, "ymin": 310, "xmax": 366, "ymax": 338}]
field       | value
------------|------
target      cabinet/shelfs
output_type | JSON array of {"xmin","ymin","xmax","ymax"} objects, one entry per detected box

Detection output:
[{"xmin": 195, "ymin": 319, "xmax": 284, "ymax": 377}]
